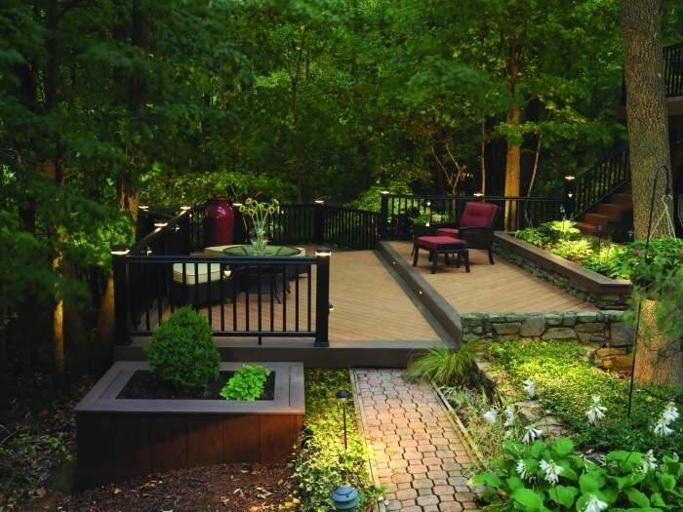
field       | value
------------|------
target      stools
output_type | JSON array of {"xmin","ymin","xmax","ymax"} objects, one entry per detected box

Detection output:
[{"xmin": 411, "ymin": 234, "xmax": 469, "ymax": 274}]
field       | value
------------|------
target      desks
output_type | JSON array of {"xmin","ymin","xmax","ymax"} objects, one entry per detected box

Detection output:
[{"xmin": 200, "ymin": 243, "xmax": 305, "ymax": 305}]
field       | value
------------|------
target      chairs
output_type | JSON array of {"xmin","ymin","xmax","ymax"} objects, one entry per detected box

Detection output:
[
  {"xmin": 428, "ymin": 199, "xmax": 501, "ymax": 269},
  {"xmin": 159, "ymin": 230, "xmax": 228, "ymax": 311}
]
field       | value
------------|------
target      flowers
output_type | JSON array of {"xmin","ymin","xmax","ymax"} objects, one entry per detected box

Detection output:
[{"xmin": 237, "ymin": 196, "xmax": 280, "ymax": 248}]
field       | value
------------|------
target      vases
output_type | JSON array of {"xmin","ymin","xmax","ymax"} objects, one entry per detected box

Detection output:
[{"xmin": 248, "ymin": 225, "xmax": 268, "ymax": 251}]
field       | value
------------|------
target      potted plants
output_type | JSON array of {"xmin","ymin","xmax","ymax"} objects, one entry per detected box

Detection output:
[
  {"xmin": 616, "ymin": 237, "xmax": 682, "ymax": 337},
  {"xmin": 70, "ymin": 301, "xmax": 305, "ymax": 492}
]
